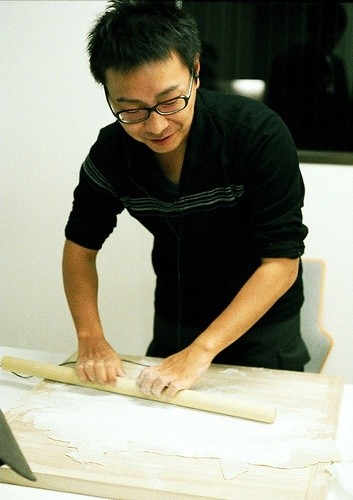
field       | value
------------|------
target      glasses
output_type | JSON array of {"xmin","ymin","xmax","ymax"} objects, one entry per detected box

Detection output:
[{"xmin": 104, "ymin": 67, "xmax": 195, "ymax": 125}]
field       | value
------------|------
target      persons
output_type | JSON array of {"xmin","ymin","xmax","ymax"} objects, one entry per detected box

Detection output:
[{"xmin": 61, "ymin": 2, "xmax": 309, "ymax": 397}]
